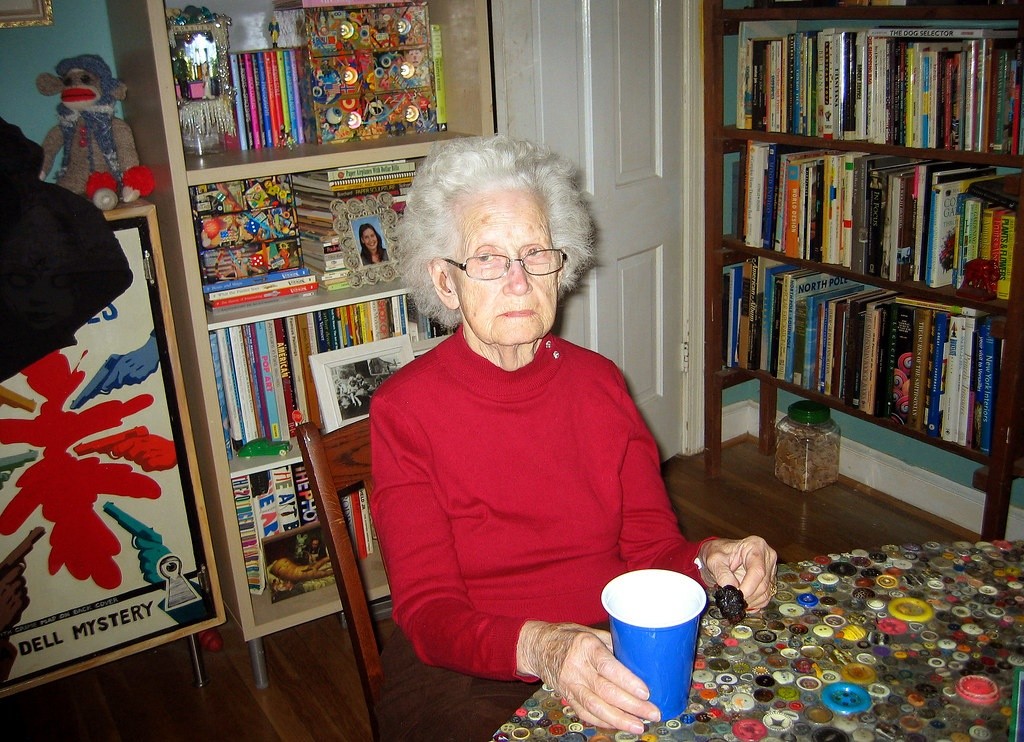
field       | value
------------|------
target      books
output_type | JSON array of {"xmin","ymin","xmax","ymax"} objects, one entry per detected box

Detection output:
[
  {"xmin": 232, "ymin": 461, "xmax": 378, "ymax": 595},
  {"xmin": 725, "ymin": 21, "xmax": 1024, "ymax": 454},
  {"xmin": 429, "ymin": 24, "xmax": 448, "ymax": 132},
  {"xmin": 223, "ymin": 49, "xmax": 306, "ymax": 150},
  {"xmin": 186, "ymin": 157, "xmax": 419, "ymax": 317},
  {"xmin": 208, "ymin": 293, "xmax": 460, "ymax": 461}
]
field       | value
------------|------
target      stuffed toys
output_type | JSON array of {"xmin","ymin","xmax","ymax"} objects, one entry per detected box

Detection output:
[{"xmin": 37, "ymin": 53, "xmax": 154, "ymax": 210}]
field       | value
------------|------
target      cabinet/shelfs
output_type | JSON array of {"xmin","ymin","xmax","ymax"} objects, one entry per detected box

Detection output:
[
  {"xmin": 700, "ymin": 0, "xmax": 1024, "ymax": 543},
  {"xmin": 0, "ymin": 199, "xmax": 229, "ymax": 698},
  {"xmin": 104, "ymin": 0, "xmax": 497, "ymax": 694}
]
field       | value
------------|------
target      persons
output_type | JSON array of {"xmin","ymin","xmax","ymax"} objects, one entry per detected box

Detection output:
[
  {"xmin": 306, "ymin": 540, "xmax": 324, "ymax": 564},
  {"xmin": 359, "ymin": 223, "xmax": 389, "ymax": 266},
  {"xmin": 370, "ymin": 135, "xmax": 777, "ymax": 734},
  {"xmin": 344, "ymin": 374, "xmax": 358, "ymax": 407},
  {"xmin": 354, "ymin": 373, "xmax": 369, "ymax": 406}
]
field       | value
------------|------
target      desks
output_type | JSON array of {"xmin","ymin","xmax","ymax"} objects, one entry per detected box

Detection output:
[{"xmin": 490, "ymin": 541, "xmax": 1024, "ymax": 742}]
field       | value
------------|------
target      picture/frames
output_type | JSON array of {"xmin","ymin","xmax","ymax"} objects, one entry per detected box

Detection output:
[
  {"xmin": 308, "ymin": 333, "xmax": 415, "ymax": 433},
  {"xmin": 0, "ymin": 0, "xmax": 55, "ymax": 28}
]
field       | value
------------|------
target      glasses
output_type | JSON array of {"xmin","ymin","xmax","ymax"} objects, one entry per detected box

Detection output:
[{"xmin": 441, "ymin": 248, "xmax": 568, "ymax": 281}]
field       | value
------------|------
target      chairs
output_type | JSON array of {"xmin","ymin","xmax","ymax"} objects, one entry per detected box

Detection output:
[{"xmin": 293, "ymin": 417, "xmax": 424, "ymax": 705}]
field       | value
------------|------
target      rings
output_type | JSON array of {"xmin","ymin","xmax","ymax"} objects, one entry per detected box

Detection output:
[{"xmin": 769, "ymin": 581, "xmax": 777, "ymax": 596}]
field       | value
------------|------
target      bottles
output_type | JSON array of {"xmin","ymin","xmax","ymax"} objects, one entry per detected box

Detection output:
[{"xmin": 774, "ymin": 402, "xmax": 841, "ymax": 492}]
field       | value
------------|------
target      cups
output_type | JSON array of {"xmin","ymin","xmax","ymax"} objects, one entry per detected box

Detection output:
[{"xmin": 601, "ymin": 569, "xmax": 709, "ymax": 721}]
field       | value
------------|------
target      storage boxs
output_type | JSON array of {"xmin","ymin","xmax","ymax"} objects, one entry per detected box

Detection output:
[{"xmin": 274, "ymin": 0, "xmax": 439, "ymax": 145}]
